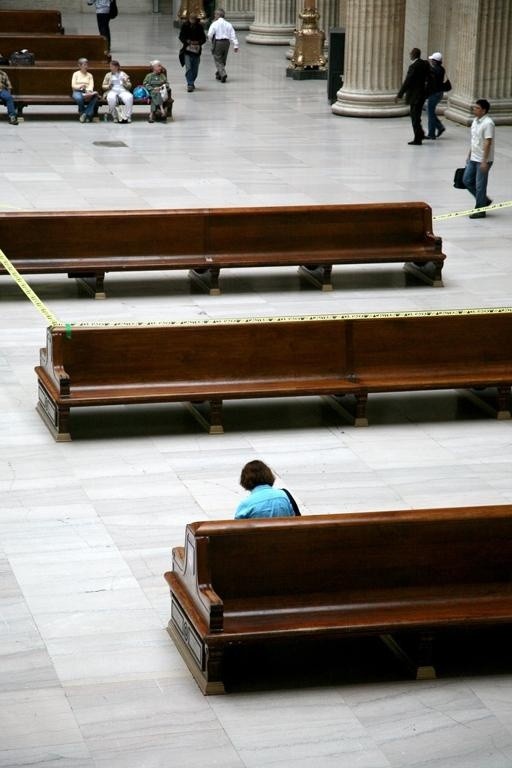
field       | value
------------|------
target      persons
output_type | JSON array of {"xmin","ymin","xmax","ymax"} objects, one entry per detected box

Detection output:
[
  {"xmin": 71, "ymin": 57, "xmax": 99, "ymax": 122},
  {"xmin": 1, "ymin": 70, "xmax": 19, "ymax": 124},
  {"xmin": 205, "ymin": 8, "xmax": 239, "ymax": 82},
  {"xmin": 87, "ymin": 1, "xmax": 118, "ymax": 53},
  {"xmin": 229, "ymin": 459, "xmax": 300, "ymax": 519},
  {"xmin": 142, "ymin": 60, "xmax": 172, "ymax": 123},
  {"xmin": 426, "ymin": 52, "xmax": 451, "ymax": 142},
  {"xmin": 175, "ymin": 9, "xmax": 203, "ymax": 94},
  {"xmin": 458, "ymin": 97, "xmax": 495, "ymax": 220},
  {"xmin": 394, "ymin": 45, "xmax": 428, "ymax": 146},
  {"xmin": 100, "ymin": 60, "xmax": 133, "ymax": 123}
]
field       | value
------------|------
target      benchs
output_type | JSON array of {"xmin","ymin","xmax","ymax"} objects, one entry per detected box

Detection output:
[
  {"xmin": 162, "ymin": 505, "xmax": 511, "ymax": 697},
  {"xmin": 0, "ymin": 200, "xmax": 446, "ymax": 298},
  {"xmin": 34, "ymin": 304, "xmax": 512, "ymax": 442},
  {"xmin": 0, "ymin": 8, "xmax": 173, "ymax": 122}
]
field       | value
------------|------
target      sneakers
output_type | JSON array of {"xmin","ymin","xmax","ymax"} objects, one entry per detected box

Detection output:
[
  {"xmin": 408, "ymin": 128, "xmax": 445, "ymax": 145},
  {"xmin": 8, "ymin": 118, "xmax": 19, "ymax": 125},
  {"xmin": 80, "ymin": 111, "xmax": 168, "ymax": 122}
]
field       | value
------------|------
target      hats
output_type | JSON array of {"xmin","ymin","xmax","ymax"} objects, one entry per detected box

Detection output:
[{"xmin": 428, "ymin": 52, "xmax": 442, "ymax": 61}]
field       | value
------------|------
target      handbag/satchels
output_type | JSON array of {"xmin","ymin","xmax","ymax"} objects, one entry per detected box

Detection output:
[
  {"xmin": 454, "ymin": 169, "xmax": 470, "ymax": 189},
  {"xmin": 442, "ymin": 80, "xmax": 451, "ymax": 92}
]
